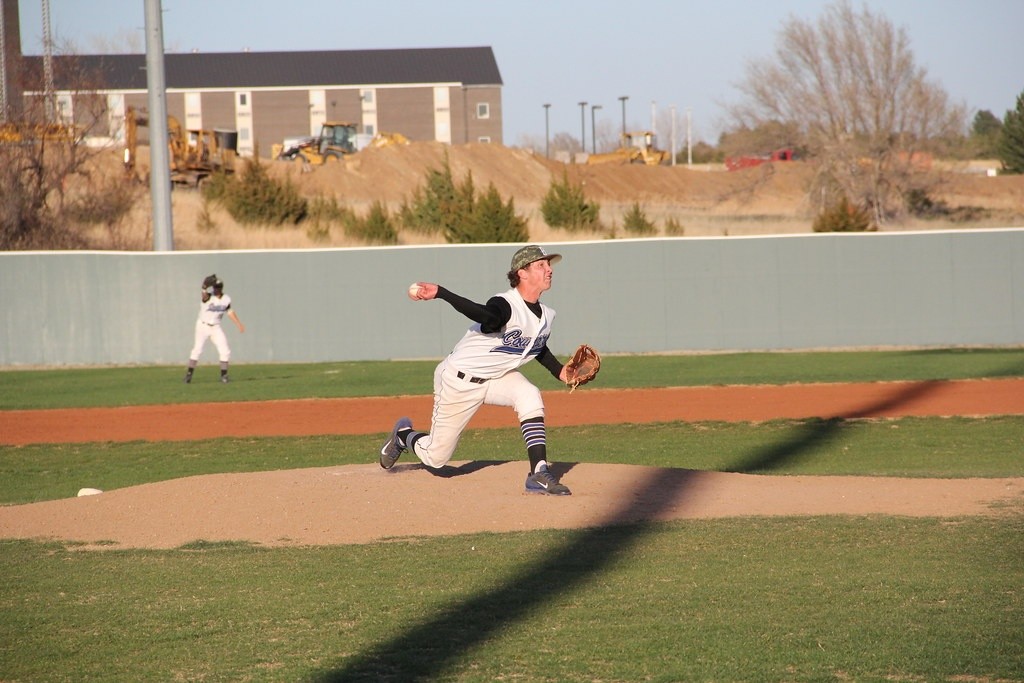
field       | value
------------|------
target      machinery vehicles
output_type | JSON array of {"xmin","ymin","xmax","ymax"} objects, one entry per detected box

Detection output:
[
  {"xmin": 269, "ymin": 119, "xmax": 410, "ymax": 171},
  {"xmin": 587, "ymin": 130, "xmax": 671, "ymax": 169},
  {"xmin": 123, "ymin": 104, "xmax": 253, "ymax": 195},
  {"xmin": 1, "ymin": 0, "xmax": 87, "ymax": 149}
]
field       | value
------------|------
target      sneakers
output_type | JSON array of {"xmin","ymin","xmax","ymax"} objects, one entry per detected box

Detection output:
[
  {"xmin": 185, "ymin": 373, "xmax": 191, "ymax": 383},
  {"xmin": 525, "ymin": 470, "xmax": 572, "ymax": 496},
  {"xmin": 380, "ymin": 417, "xmax": 413, "ymax": 469},
  {"xmin": 221, "ymin": 375, "xmax": 229, "ymax": 383}
]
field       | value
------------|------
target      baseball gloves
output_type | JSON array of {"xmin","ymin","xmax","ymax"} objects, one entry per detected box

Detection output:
[
  {"xmin": 202, "ymin": 274, "xmax": 217, "ymax": 289},
  {"xmin": 565, "ymin": 345, "xmax": 601, "ymax": 386}
]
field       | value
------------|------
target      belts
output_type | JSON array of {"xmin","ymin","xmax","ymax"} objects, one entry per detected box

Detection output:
[
  {"xmin": 457, "ymin": 371, "xmax": 489, "ymax": 384},
  {"xmin": 202, "ymin": 322, "xmax": 214, "ymax": 326}
]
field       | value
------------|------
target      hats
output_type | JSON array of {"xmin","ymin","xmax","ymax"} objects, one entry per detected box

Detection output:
[
  {"xmin": 511, "ymin": 245, "xmax": 562, "ymax": 274},
  {"xmin": 213, "ymin": 280, "xmax": 222, "ymax": 286}
]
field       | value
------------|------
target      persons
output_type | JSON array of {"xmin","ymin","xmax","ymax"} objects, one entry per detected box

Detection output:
[
  {"xmin": 184, "ymin": 275, "xmax": 245, "ymax": 383},
  {"xmin": 379, "ymin": 245, "xmax": 601, "ymax": 496}
]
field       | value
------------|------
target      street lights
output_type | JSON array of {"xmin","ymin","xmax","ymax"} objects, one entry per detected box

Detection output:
[
  {"xmin": 618, "ymin": 96, "xmax": 633, "ymax": 132},
  {"xmin": 578, "ymin": 102, "xmax": 589, "ymax": 152},
  {"xmin": 591, "ymin": 105, "xmax": 603, "ymax": 155},
  {"xmin": 542, "ymin": 104, "xmax": 554, "ymax": 160}
]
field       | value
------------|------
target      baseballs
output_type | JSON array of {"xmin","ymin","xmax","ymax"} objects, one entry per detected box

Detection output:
[{"xmin": 409, "ymin": 283, "xmax": 424, "ymax": 297}]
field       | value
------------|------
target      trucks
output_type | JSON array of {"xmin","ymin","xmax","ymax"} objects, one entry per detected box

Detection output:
[{"xmin": 725, "ymin": 146, "xmax": 802, "ymax": 171}]
